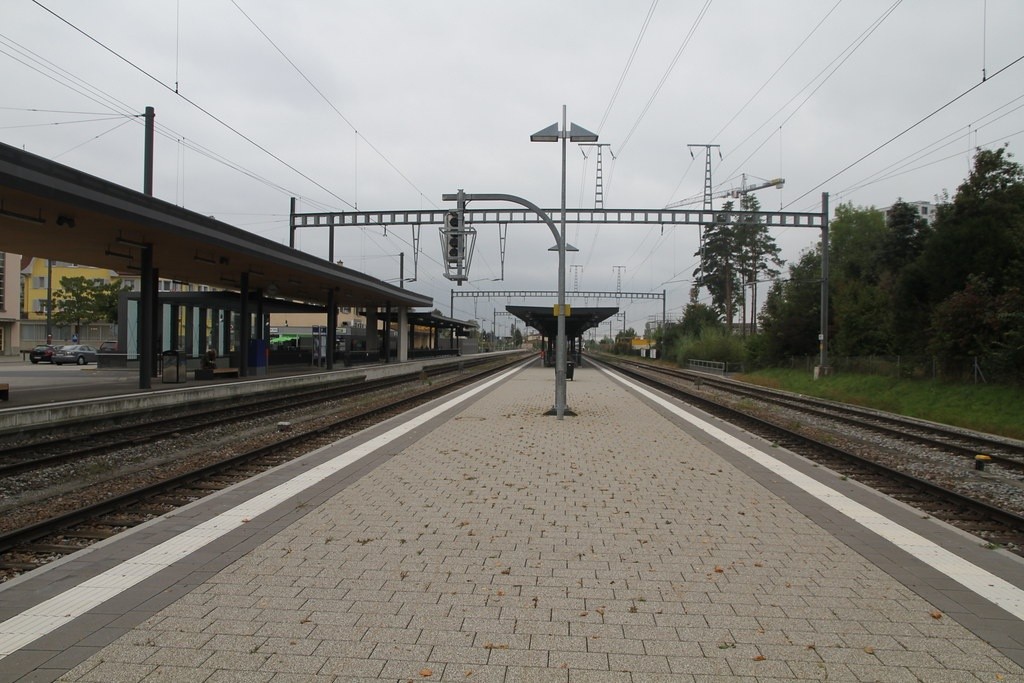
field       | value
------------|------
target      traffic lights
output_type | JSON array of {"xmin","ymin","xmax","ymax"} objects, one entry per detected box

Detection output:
[{"xmin": 444, "ymin": 213, "xmax": 465, "ymax": 261}]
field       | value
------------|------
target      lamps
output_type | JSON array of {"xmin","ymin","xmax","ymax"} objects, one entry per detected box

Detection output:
[
  {"xmin": 287, "ymin": 274, "xmax": 302, "ymax": 285},
  {"xmin": 172, "ymin": 278, "xmax": 189, "ymax": 285},
  {"xmin": 116, "ymin": 229, "xmax": 148, "ymax": 249},
  {"xmin": 126, "ymin": 260, "xmax": 141, "ymax": 272},
  {"xmin": 193, "ymin": 249, "xmax": 216, "ymax": 266},
  {"xmin": 219, "ymin": 272, "xmax": 236, "ymax": 283},
  {"xmin": 105, "ymin": 243, "xmax": 133, "ymax": 261},
  {"xmin": 0, "ymin": 200, "xmax": 46, "ymax": 226}
]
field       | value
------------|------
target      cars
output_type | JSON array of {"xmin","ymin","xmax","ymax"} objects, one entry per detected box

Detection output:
[
  {"xmin": 51, "ymin": 344, "xmax": 98, "ymax": 365},
  {"xmin": 99, "ymin": 341, "xmax": 119, "ymax": 353},
  {"xmin": 30, "ymin": 344, "xmax": 63, "ymax": 364}
]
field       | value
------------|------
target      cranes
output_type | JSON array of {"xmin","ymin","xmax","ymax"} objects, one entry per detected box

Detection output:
[{"xmin": 664, "ymin": 172, "xmax": 787, "ymax": 210}]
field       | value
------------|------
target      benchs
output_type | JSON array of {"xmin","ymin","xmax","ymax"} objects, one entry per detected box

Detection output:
[
  {"xmin": 194, "ymin": 367, "xmax": 239, "ymax": 380},
  {"xmin": 0, "ymin": 383, "xmax": 9, "ymax": 401}
]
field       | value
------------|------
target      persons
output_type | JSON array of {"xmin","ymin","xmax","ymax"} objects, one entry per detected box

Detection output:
[{"xmin": 200, "ymin": 350, "xmax": 216, "ymax": 369}]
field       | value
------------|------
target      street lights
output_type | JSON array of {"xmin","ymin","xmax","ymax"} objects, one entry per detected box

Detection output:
[{"xmin": 529, "ymin": 104, "xmax": 600, "ymax": 415}]
